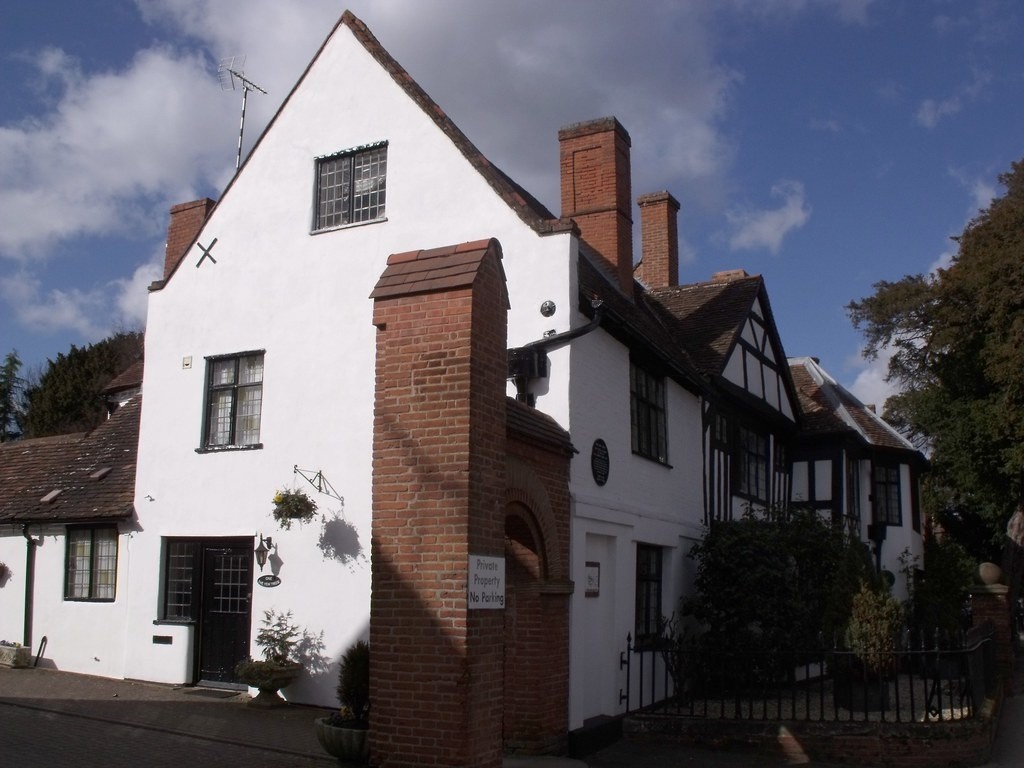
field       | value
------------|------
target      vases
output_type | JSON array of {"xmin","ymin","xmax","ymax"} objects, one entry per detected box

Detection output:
[{"xmin": 0, "ymin": 646, "xmax": 31, "ymax": 668}]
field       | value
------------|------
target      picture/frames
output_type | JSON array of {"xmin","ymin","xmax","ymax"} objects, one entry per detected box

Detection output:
[{"xmin": 585, "ymin": 561, "xmax": 600, "ymax": 598}]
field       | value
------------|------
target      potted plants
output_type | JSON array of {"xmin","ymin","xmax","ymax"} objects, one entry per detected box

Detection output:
[
  {"xmin": 833, "ymin": 577, "xmax": 910, "ymax": 712},
  {"xmin": 234, "ymin": 608, "xmax": 301, "ymax": 710},
  {"xmin": 315, "ymin": 639, "xmax": 370, "ymax": 768}
]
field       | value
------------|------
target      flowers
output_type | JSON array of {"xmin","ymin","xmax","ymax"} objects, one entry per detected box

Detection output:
[
  {"xmin": 270, "ymin": 485, "xmax": 320, "ymax": 531},
  {"xmin": 0, "ymin": 640, "xmax": 21, "ymax": 648}
]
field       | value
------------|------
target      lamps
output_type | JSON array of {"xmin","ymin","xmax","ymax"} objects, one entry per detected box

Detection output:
[{"xmin": 256, "ymin": 535, "xmax": 271, "ymax": 572}]
